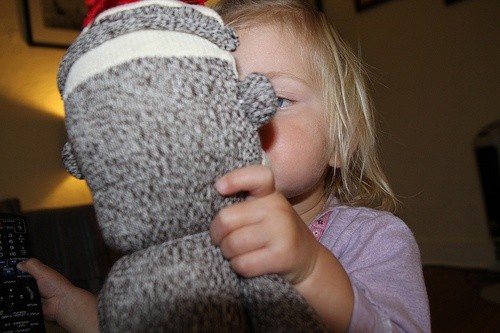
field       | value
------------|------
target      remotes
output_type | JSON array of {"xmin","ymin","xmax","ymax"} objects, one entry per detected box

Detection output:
[{"xmin": 0, "ymin": 198, "xmax": 45, "ymax": 333}]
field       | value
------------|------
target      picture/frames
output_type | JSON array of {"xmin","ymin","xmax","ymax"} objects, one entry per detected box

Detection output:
[{"xmin": 23, "ymin": 1, "xmax": 86, "ymax": 50}]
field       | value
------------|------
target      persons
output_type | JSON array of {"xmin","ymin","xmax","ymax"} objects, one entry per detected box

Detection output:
[{"xmin": 16, "ymin": 1, "xmax": 431, "ymax": 333}]
